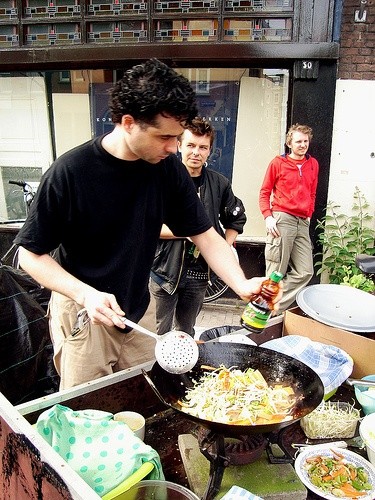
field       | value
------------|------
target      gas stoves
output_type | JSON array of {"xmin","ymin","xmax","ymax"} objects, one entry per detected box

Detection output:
[{"xmin": 197, "ymin": 424, "xmax": 270, "ymax": 465}]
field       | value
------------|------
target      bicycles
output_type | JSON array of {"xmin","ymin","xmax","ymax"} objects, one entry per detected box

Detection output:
[
  {"xmin": 0, "ymin": 176, "xmax": 61, "ymax": 274},
  {"xmin": 202, "ymin": 263, "xmax": 228, "ymax": 304}
]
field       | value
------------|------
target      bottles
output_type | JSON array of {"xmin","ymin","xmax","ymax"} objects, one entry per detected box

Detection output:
[{"xmin": 239, "ymin": 270, "xmax": 283, "ymax": 334}]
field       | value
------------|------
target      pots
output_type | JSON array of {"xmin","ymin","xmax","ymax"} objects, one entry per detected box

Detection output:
[{"xmin": 141, "ymin": 342, "xmax": 325, "ymax": 436}]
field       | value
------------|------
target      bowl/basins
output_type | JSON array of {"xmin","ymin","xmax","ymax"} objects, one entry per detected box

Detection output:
[
  {"xmin": 112, "ymin": 479, "xmax": 201, "ymax": 500},
  {"xmin": 354, "ymin": 375, "xmax": 375, "ymax": 415},
  {"xmin": 359, "ymin": 413, "xmax": 375, "ymax": 465},
  {"xmin": 300, "ymin": 401, "xmax": 360, "ymax": 440},
  {"xmin": 102, "ymin": 462, "xmax": 156, "ymax": 500}
]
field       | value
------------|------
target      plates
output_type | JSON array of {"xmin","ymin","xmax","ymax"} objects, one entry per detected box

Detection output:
[{"xmin": 294, "ymin": 447, "xmax": 375, "ymax": 500}]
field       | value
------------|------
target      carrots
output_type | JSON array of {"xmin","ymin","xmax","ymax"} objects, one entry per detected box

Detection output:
[{"xmin": 307, "ymin": 448, "xmax": 369, "ymax": 498}]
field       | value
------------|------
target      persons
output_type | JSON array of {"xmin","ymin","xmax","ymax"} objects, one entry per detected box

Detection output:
[
  {"xmin": 16, "ymin": 57, "xmax": 283, "ymax": 393},
  {"xmin": 150, "ymin": 120, "xmax": 247, "ymax": 340},
  {"xmin": 257, "ymin": 123, "xmax": 319, "ymax": 315}
]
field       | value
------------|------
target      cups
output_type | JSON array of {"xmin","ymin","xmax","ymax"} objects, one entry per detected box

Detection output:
[{"xmin": 113, "ymin": 411, "xmax": 146, "ymax": 441}]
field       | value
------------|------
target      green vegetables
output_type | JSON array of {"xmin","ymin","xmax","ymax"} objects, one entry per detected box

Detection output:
[{"xmin": 308, "ymin": 461, "xmax": 372, "ymax": 490}]
